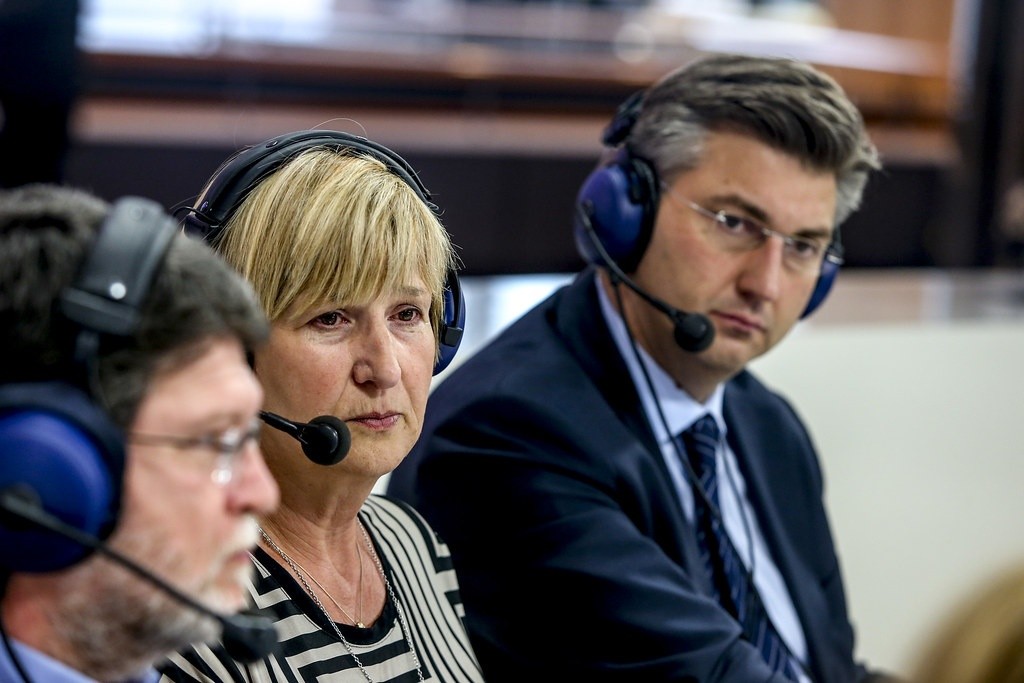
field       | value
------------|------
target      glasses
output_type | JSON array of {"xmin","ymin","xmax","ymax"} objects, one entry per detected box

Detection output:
[
  {"xmin": 657, "ymin": 180, "xmax": 828, "ymax": 279},
  {"xmin": 122, "ymin": 419, "xmax": 261, "ymax": 487}
]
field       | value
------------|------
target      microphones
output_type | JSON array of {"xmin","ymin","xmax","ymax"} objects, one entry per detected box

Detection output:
[
  {"xmin": 257, "ymin": 411, "xmax": 350, "ymax": 466},
  {"xmin": 586, "ymin": 228, "xmax": 716, "ymax": 354},
  {"xmin": 42, "ymin": 515, "xmax": 278, "ymax": 665}
]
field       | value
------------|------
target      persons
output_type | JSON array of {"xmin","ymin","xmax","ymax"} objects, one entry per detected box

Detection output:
[
  {"xmin": 384, "ymin": 52, "xmax": 906, "ymax": 683},
  {"xmin": 0, "ymin": 186, "xmax": 280, "ymax": 683},
  {"xmin": 150, "ymin": 130, "xmax": 488, "ymax": 683}
]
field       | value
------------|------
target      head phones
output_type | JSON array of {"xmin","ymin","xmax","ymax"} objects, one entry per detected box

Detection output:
[
  {"xmin": 571, "ymin": 91, "xmax": 846, "ymax": 321},
  {"xmin": 0, "ymin": 196, "xmax": 184, "ymax": 577},
  {"xmin": 173, "ymin": 127, "xmax": 467, "ymax": 378}
]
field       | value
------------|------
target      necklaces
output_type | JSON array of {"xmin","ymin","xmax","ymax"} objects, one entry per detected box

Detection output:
[{"xmin": 256, "ymin": 517, "xmax": 425, "ymax": 683}]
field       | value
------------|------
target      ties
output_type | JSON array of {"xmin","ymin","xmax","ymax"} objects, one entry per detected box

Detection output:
[{"xmin": 676, "ymin": 413, "xmax": 805, "ymax": 683}]
